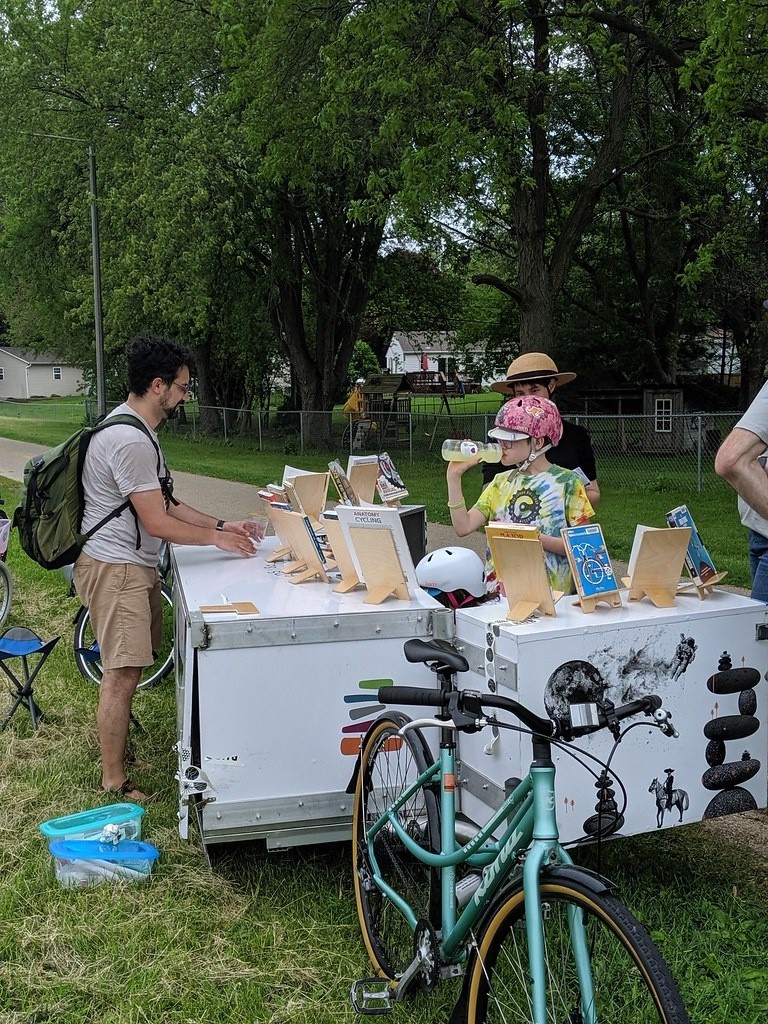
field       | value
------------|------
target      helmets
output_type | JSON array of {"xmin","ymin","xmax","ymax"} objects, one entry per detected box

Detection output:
[
  {"xmin": 415, "ymin": 546, "xmax": 487, "ymax": 598},
  {"xmin": 488, "ymin": 395, "xmax": 563, "ymax": 446}
]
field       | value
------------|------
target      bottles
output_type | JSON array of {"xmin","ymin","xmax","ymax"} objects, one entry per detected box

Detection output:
[{"xmin": 441, "ymin": 439, "xmax": 503, "ymax": 464}]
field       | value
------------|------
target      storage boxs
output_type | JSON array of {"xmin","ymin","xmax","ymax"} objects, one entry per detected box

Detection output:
[
  {"xmin": 48, "ymin": 840, "xmax": 160, "ymax": 889},
  {"xmin": 38, "ymin": 801, "xmax": 146, "ymax": 845}
]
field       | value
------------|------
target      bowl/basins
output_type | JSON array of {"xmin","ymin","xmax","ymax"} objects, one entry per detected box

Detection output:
[
  {"xmin": 49, "ymin": 839, "xmax": 160, "ymax": 887},
  {"xmin": 41, "ymin": 802, "xmax": 144, "ymax": 854}
]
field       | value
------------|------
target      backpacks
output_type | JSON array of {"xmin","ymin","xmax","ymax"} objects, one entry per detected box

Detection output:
[{"xmin": 12, "ymin": 414, "xmax": 160, "ymax": 572}]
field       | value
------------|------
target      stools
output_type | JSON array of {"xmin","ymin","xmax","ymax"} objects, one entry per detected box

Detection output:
[
  {"xmin": 0, "ymin": 626, "xmax": 62, "ymax": 736},
  {"xmin": 75, "ymin": 638, "xmax": 159, "ymax": 736}
]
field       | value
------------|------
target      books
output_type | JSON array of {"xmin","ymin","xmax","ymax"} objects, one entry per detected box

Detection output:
[
  {"xmin": 666, "ymin": 504, "xmax": 717, "ymax": 587},
  {"xmin": 484, "ymin": 521, "xmax": 555, "ymax": 601},
  {"xmin": 563, "ymin": 523, "xmax": 618, "ymax": 599},
  {"xmin": 257, "ymin": 452, "xmax": 409, "ymax": 572},
  {"xmin": 334, "ymin": 505, "xmax": 419, "ymax": 589}
]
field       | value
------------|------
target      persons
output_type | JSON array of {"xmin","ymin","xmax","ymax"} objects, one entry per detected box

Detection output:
[
  {"xmin": 72, "ymin": 337, "xmax": 265, "ymax": 802},
  {"xmin": 482, "ymin": 352, "xmax": 600, "ymax": 505},
  {"xmin": 714, "ymin": 380, "xmax": 768, "ymax": 602},
  {"xmin": 446, "ymin": 396, "xmax": 596, "ymax": 596}
]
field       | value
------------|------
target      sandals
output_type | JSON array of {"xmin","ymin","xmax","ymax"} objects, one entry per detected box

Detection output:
[{"xmin": 100, "ymin": 780, "xmax": 150, "ymax": 805}]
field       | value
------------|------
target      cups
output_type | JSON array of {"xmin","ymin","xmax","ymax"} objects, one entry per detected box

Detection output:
[{"xmin": 243, "ymin": 513, "xmax": 268, "ymax": 547}]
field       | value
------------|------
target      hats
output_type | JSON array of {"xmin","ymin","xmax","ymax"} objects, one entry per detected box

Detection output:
[{"xmin": 490, "ymin": 351, "xmax": 577, "ymax": 394}]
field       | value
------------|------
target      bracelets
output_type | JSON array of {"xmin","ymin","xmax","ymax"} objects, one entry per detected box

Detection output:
[
  {"xmin": 447, "ymin": 497, "xmax": 465, "ymax": 509},
  {"xmin": 216, "ymin": 520, "xmax": 226, "ymax": 531}
]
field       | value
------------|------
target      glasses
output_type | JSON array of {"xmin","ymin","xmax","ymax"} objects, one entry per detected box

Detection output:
[
  {"xmin": 497, "ymin": 437, "xmax": 513, "ymax": 450},
  {"xmin": 174, "ymin": 381, "xmax": 190, "ymax": 395}
]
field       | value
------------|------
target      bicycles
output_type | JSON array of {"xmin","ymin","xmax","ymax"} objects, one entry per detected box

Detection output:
[
  {"xmin": 338, "ymin": 639, "xmax": 696, "ymax": 1024},
  {"xmin": 0, "ymin": 498, "xmax": 176, "ymax": 692}
]
